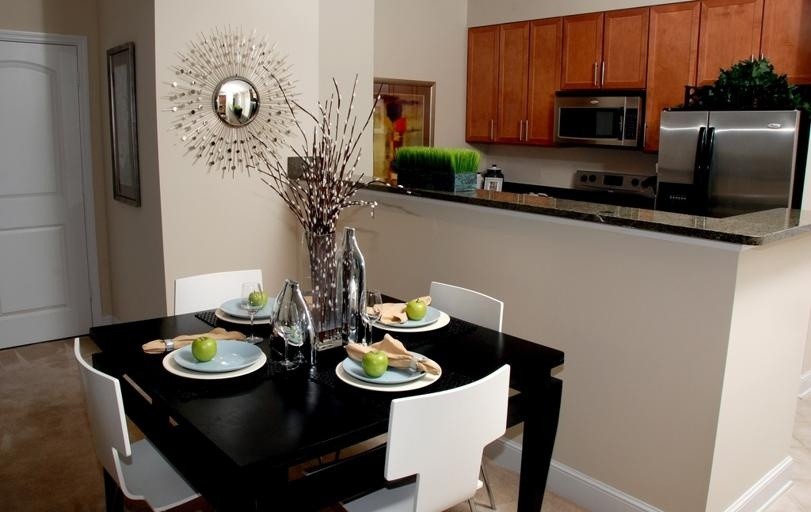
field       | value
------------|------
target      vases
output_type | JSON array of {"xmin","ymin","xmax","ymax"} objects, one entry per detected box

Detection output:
[{"xmin": 302, "ymin": 229, "xmax": 349, "ymax": 353}]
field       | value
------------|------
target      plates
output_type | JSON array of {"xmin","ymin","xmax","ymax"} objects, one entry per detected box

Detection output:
[
  {"xmin": 362, "ymin": 304, "xmax": 449, "ymax": 334},
  {"xmin": 160, "ymin": 340, "xmax": 267, "ymax": 379},
  {"xmin": 335, "ymin": 352, "xmax": 442, "ymax": 392},
  {"xmin": 213, "ymin": 297, "xmax": 277, "ymax": 324}
]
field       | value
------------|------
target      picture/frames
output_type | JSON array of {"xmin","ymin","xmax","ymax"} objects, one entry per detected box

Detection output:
[{"xmin": 105, "ymin": 40, "xmax": 142, "ymax": 209}]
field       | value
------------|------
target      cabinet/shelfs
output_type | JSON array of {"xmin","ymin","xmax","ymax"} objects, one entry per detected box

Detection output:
[
  {"xmin": 644, "ymin": 0, "xmax": 701, "ymax": 152},
  {"xmin": 696, "ymin": 0, "xmax": 810, "ymax": 87},
  {"xmin": 464, "ymin": 25, "xmax": 498, "ymax": 144},
  {"xmin": 562, "ymin": 5, "xmax": 646, "ymax": 92},
  {"xmin": 498, "ymin": 16, "xmax": 562, "ymax": 147}
]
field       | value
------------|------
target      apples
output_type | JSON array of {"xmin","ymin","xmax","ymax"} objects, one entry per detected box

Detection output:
[
  {"xmin": 191, "ymin": 335, "xmax": 217, "ymax": 363},
  {"xmin": 406, "ymin": 297, "xmax": 427, "ymax": 321},
  {"xmin": 360, "ymin": 349, "xmax": 388, "ymax": 379},
  {"xmin": 248, "ymin": 290, "xmax": 268, "ymax": 307}
]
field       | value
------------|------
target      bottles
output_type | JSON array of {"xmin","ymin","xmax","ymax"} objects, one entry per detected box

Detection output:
[
  {"xmin": 336, "ymin": 226, "xmax": 366, "ymax": 345},
  {"xmin": 272, "ymin": 279, "xmax": 318, "ymax": 369}
]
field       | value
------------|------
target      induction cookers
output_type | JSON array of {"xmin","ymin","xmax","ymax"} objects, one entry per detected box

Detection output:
[{"xmin": 571, "ymin": 191, "xmax": 655, "ymax": 208}]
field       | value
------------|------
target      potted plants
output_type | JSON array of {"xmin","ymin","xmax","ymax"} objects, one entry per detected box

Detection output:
[{"xmin": 391, "ymin": 146, "xmax": 480, "ymax": 193}]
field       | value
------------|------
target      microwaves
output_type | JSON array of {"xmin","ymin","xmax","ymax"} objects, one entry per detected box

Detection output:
[{"xmin": 557, "ymin": 94, "xmax": 645, "ymax": 149}]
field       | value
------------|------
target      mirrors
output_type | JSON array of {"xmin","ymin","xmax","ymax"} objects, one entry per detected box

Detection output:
[{"xmin": 210, "ymin": 77, "xmax": 262, "ymax": 130}]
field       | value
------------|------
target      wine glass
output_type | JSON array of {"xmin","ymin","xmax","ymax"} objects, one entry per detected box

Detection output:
[
  {"xmin": 358, "ymin": 288, "xmax": 383, "ymax": 345},
  {"xmin": 274, "ymin": 301, "xmax": 299, "ymax": 373},
  {"xmin": 239, "ymin": 281, "xmax": 265, "ymax": 344}
]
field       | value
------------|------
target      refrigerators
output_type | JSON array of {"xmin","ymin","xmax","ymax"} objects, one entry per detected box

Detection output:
[{"xmin": 655, "ymin": 107, "xmax": 800, "ymax": 215}]
